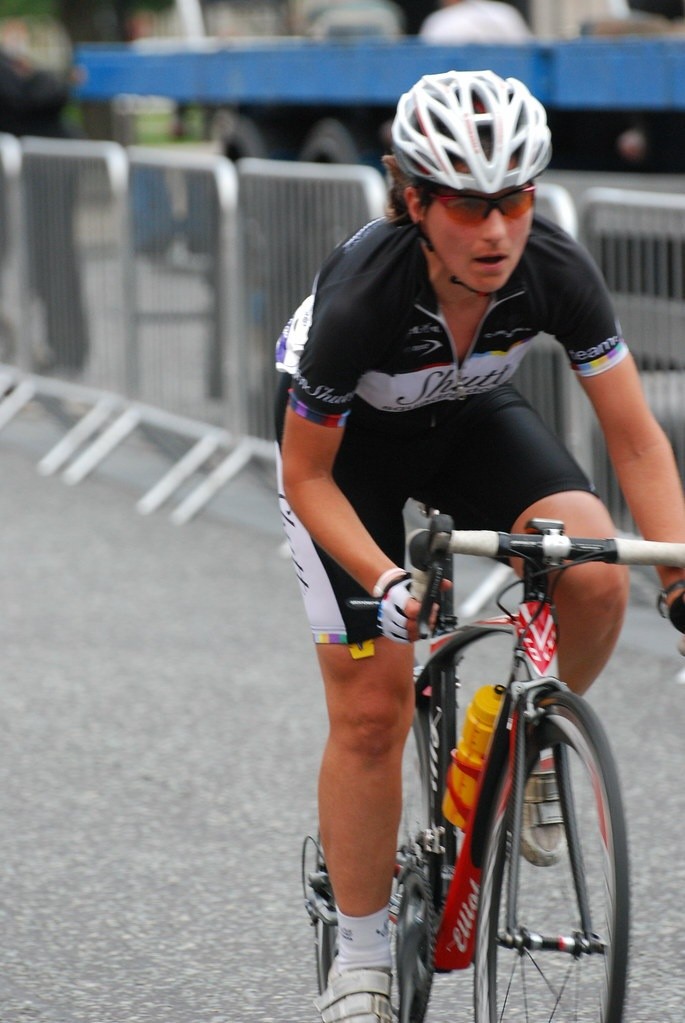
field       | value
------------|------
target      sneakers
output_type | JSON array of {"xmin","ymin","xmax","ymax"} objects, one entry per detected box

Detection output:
[
  {"xmin": 315, "ymin": 955, "xmax": 393, "ymax": 1023},
  {"xmin": 521, "ymin": 747, "xmax": 568, "ymax": 865}
]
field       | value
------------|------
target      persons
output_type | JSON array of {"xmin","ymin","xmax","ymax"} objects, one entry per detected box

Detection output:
[
  {"xmin": 0, "ymin": 1, "xmax": 685, "ymax": 432},
  {"xmin": 274, "ymin": 69, "xmax": 685, "ymax": 1023}
]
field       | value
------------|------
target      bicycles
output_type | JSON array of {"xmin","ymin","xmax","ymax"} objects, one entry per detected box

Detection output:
[{"xmin": 301, "ymin": 497, "xmax": 685, "ymax": 1023}]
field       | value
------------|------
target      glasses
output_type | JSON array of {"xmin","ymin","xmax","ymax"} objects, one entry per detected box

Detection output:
[{"xmin": 425, "ymin": 186, "xmax": 534, "ymax": 228}]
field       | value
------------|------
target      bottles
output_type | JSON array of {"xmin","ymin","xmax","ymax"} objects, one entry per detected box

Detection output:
[{"xmin": 440, "ymin": 685, "xmax": 509, "ymax": 828}]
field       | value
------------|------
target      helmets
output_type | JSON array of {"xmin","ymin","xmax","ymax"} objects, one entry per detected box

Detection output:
[{"xmin": 391, "ymin": 68, "xmax": 551, "ymax": 194}]
